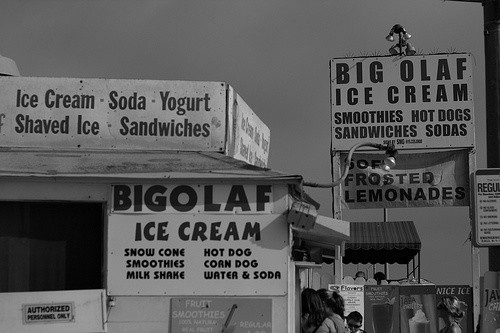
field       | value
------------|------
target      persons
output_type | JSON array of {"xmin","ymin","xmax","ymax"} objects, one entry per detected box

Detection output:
[
  {"xmin": 355, "ymin": 270, "xmax": 365, "ymax": 279},
  {"xmin": 374, "ymin": 272, "xmax": 385, "ymax": 284},
  {"xmin": 346, "ymin": 312, "xmax": 362, "ymax": 333},
  {"xmin": 300, "ymin": 287, "xmax": 346, "ymax": 333}
]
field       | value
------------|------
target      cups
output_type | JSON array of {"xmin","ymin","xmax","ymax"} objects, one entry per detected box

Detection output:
[
  {"xmin": 217, "ymin": 321, "xmax": 240, "ymax": 333},
  {"xmin": 487, "ymin": 300, "xmax": 499, "ymax": 324},
  {"xmin": 371, "ymin": 302, "xmax": 395, "ymax": 333},
  {"xmin": 409, "ymin": 319, "xmax": 431, "ymax": 333}
]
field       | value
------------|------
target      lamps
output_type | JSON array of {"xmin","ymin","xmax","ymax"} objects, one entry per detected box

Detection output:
[{"xmin": 384, "ymin": 140, "xmax": 398, "ymax": 167}]
